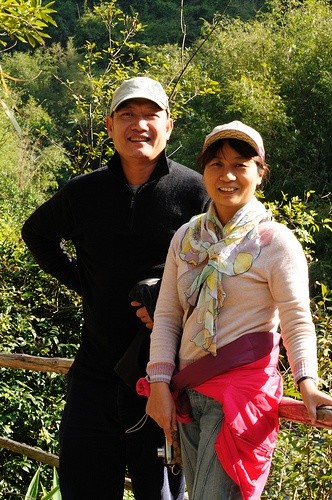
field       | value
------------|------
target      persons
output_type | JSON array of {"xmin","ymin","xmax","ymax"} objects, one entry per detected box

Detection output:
[
  {"xmin": 22, "ymin": 78, "xmax": 211, "ymax": 498},
  {"xmin": 145, "ymin": 123, "xmax": 332, "ymax": 500}
]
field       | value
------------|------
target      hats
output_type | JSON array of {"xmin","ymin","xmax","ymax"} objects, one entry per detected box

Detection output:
[
  {"xmin": 202, "ymin": 121, "xmax": 265, "ymax": 161},
  {"xmin": 110, "ymin": 78, "xmax": 170, "ymax": 116}
]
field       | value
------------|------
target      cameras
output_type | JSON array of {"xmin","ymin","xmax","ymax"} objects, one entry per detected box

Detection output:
[{"xmin": 158, "ymin": 438, "xmax": 174, "ymax": 464}]
